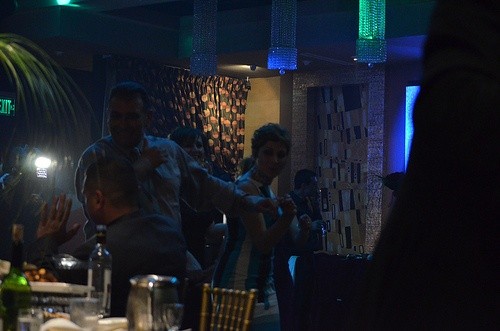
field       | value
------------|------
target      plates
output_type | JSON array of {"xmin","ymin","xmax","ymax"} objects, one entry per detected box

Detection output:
[{"xmin": 29, "ymin": 281, "xmax": 97, "ymax": 295}]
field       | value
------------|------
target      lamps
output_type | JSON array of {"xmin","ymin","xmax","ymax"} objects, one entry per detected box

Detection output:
[
  {"xmin": 267, "ymin": 0, "xmax": 298, "ymax": 76},
  {"xmin": 190, "ymin": 0, "xmax": 218, "ymax": 77},
  {"xmin": 355, "ymin": 0, "xmax": 387, "ymax": 69}
]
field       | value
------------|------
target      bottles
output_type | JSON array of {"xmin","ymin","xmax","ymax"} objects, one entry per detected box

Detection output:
[
  {"xmin": 84, "ymin": 224, "xmax": 112, "ymax": 319},
  {"xmin": 0, "ymin": 224, "xmax": 32, "ymax": 331}
]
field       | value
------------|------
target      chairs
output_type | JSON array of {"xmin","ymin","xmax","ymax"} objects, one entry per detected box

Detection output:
[{"xmin": 199, "ymin": 283, "xmax": 259, "ymax": 331}]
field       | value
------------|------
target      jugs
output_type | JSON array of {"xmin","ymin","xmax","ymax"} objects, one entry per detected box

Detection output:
[{"xmin": 124, "ymin": 275, "xmax": 179, "ymax": 331}]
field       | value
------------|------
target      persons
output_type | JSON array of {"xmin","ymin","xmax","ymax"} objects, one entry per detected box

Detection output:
[
  {"xmin": 289, "ymin": 168, "xmax": 325, "ymax": 254},
  {"xmin": 33, "ymin": 157, "xmax": 203, "ymax": 331},
  {"xmin": 75, "ymin": 82, "xmax": 286, "ymax": 258},
  {"xmin": 199, "ymin": 133, "xmax": 233, "ymax": 225},
  {"xmin": 0, "ymin": 159, "xmax": 43, "ymax": 265},
  {"xmin": 166, "ymin": 126, "xmax": 235, "ymax": 268},
  {"xmin": 208, "ymin": 122, "xmax": 295, "ymax": 331}
]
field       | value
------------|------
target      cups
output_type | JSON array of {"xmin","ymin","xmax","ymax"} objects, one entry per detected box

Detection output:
[
  {"xmin": 162, "ymin": 304, "xmax": 185, "ymax": 331},
  {"xmin": 70, "ymin": 298, "xmax": 101, "ymax": 331},
  {"xmin": 18, "ymin": 308, "xmax": 43, "ymax": 331}
]
